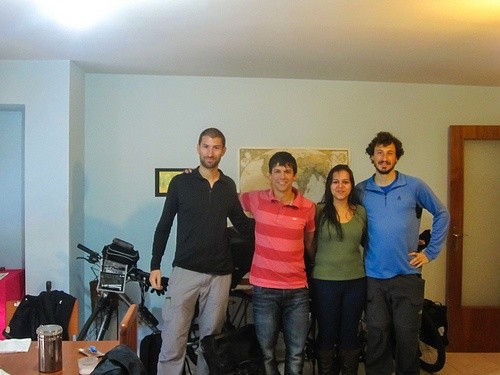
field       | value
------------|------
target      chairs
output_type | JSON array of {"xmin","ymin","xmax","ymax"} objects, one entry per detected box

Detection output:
[
  {"xmin": 120, "ymin": 304, "xmax": 138, "ymax": 354},
  {"xmin": 6, "ymin": 299, "xmax": 79, "ymax": 341}
]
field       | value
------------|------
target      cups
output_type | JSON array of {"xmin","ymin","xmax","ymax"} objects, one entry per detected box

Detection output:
[
  {"xmin": 37, "ymin": 325, "xmax": 63, "ymax": 373},
  {"xmin": 78, "ymin": 357, "xmax": 99, "ymax": 375}
]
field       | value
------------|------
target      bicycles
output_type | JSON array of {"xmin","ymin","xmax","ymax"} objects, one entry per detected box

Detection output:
[{"xmin": 75, "ymin": 238, "xmax": 446, "ymax": 375}]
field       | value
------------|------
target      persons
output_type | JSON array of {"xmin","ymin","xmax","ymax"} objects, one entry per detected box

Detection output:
[
  {"xmin": 150, "ymin": 128, "xmax": 256, "ymax": 375},
  {"xmin": 306, "ymin": 165, "xmax": 367, "ymax": 375},
  {"xmin": 182, "ymin": 152, "xmax": 317, "ymax": 375},
  {"xmin": 348, "ymin": 132, "xmax": 449, "ymax": 375}
]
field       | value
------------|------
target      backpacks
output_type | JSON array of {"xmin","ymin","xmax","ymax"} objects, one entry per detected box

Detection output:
[
  {"xmin": 139, "ymin": 333, "xmax": 194, "ymax": 375},
  {"xmin": 88, "ymin": 343, "xmax": 149, "ymax": 375}
]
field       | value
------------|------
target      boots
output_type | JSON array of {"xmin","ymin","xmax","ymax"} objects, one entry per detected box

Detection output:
[
  {"xmin": 339, "ymin": 348, "xmax": 360, "ymax": 375},
  {"xmin": 315, "ymin": 348, "xmax": 338, "ymax": 375}
]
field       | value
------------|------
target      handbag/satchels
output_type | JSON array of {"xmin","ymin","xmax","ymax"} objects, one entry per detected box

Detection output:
[
  {"xmin": 200, "ymin": 323, "xmax": 267, "ymax": 375},
  {"xmin": 420, "ymin": 298, "xmax": 449, "ymax": 349}
]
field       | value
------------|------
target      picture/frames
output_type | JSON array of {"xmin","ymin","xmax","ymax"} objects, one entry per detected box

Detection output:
[{"xmin": 155, "ymin": 168, "xmax": 187, "ymax": 198}]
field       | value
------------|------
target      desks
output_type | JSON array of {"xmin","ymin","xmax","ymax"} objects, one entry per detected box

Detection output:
[{"xmin": 0, "ymin": 340, "xmax": 120, "ymax": 375}]
